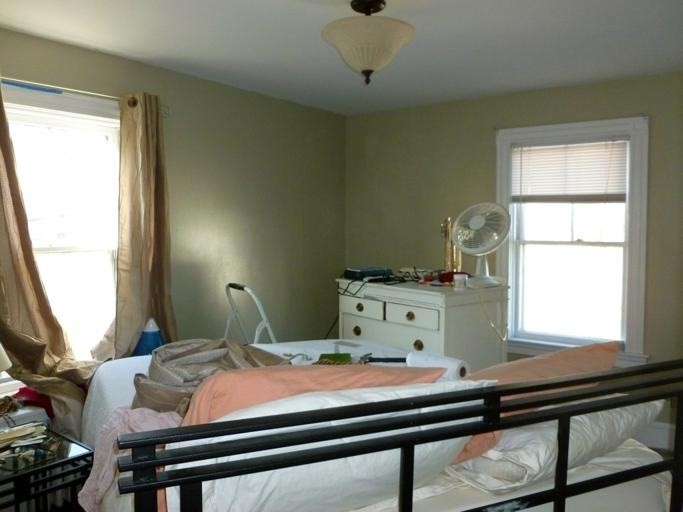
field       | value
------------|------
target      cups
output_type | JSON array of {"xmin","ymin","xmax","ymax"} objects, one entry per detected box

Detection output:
[{"xmin": 454, "ymin": 274, "xmax": 468, "ymax": 291}]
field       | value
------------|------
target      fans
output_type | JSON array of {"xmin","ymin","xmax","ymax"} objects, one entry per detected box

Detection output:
[{"xmin": 451, "ymin": 200, "xmax": 511, "ymax": 289}]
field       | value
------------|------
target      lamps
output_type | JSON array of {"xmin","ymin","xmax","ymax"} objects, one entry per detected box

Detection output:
[{"xmin": 320, "ymin": 0, "xmax": 419, "ymax": 85}]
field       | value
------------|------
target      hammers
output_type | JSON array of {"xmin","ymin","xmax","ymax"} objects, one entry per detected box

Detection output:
[{"xmin": 358, "ymin": 352, "xmax": 406, "ymax": 365}]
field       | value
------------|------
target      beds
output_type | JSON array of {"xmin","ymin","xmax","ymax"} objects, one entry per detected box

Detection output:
[{"xmin": 71, "ymin": 336, "xmax": 683, "ymax": 511}]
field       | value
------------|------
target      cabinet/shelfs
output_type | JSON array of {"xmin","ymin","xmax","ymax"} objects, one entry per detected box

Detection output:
[{"xmin": 334, "ymin": 268, "xmax": 509, "ymax": 367}]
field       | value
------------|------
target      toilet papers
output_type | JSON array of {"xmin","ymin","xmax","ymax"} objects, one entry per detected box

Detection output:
[{"xmin": 406, "ymin": 351, "xmax": 470, "ymax": 382}]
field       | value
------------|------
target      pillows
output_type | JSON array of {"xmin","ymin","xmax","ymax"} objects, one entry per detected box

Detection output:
[
  {"xmin": 447, "ymin": 396, "xmax": 662, "ymax": 495},
  {"xmin": 177, "ymin": 363, "xmax": 445, "ymax": 425},
  {"xmin": 447, "ymin": 337, "xmax": 627, "ymax": 465},
  {"xmin": 164, "ymin": 379, "xmax": 490, "ymax": 507}
]
